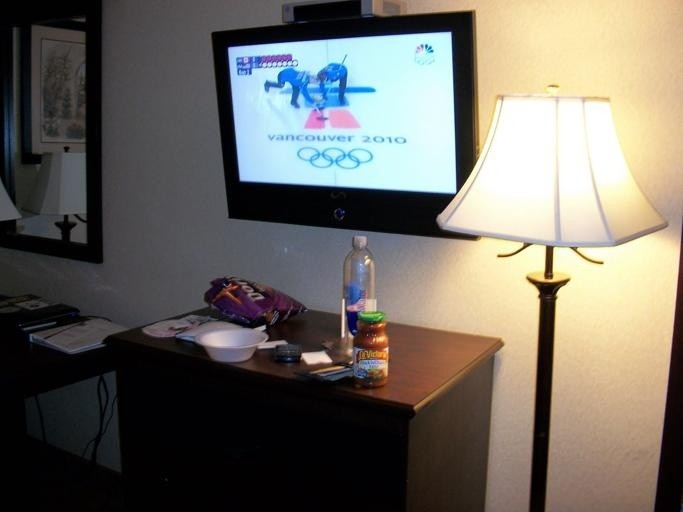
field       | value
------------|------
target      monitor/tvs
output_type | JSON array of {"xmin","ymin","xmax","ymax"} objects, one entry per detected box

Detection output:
[{"xmin": 211, "ymin": 10, "xmax": 481, "ymax": 241}]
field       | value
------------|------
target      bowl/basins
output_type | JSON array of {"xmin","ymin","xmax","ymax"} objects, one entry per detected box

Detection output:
[{"xmin": 194, "ymin": 329, "xmax": 268, "ymax": 363}]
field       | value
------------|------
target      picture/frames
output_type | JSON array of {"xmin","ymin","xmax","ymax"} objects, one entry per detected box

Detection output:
[{"xmin": 20, "ymin": 20, "xmax": 87, "ymax": 164}]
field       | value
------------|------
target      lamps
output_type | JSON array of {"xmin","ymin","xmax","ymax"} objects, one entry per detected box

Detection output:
[
  {"xmin": 21, "ymin": 146, "xmax": 86, "ymax": 241},
  {"xmin": 437, "ymin": 92, "xmax": 671, "ymax": 512},
  {"xmin": 0, "ymin": 175, "xmax": 21, "ymax": 223}
]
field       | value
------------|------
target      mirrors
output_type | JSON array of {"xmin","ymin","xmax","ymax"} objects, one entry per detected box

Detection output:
[{"xmin": 0, "ymin": 0, "xmax": 103, "ymax": 265}]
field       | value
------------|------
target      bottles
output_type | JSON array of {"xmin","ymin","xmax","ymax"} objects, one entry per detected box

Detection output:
[
  {"xmin": 341, "ymin": 235, "xmax": 376, "ymax": 340},
  {"xmin": 353, "ymin": 311, "xmax": 389, "ymax": 387}
]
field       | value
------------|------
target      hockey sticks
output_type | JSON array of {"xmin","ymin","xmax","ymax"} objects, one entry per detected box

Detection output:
[
  {"xmin": 318, "ymin": 54, "xmax": 348, "ymax": 107},
  {"xmin": 310, "ymin": 103, "xmax": 328, "ymax": 120}
]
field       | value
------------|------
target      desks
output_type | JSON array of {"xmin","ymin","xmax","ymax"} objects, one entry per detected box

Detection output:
[{"xmin": 1, "ymin": 291, "xmax": 129, "ymax": 512}]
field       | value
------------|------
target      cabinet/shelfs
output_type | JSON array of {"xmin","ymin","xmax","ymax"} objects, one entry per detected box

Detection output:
[{"xmin": 108, "ymin": 303, "xmax": 503, "ymax": 512}]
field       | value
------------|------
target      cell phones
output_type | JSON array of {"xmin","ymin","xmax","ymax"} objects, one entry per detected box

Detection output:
[{"xmin": 275, "ymin": 344, "xmax": 302, "ymax": 362}]
